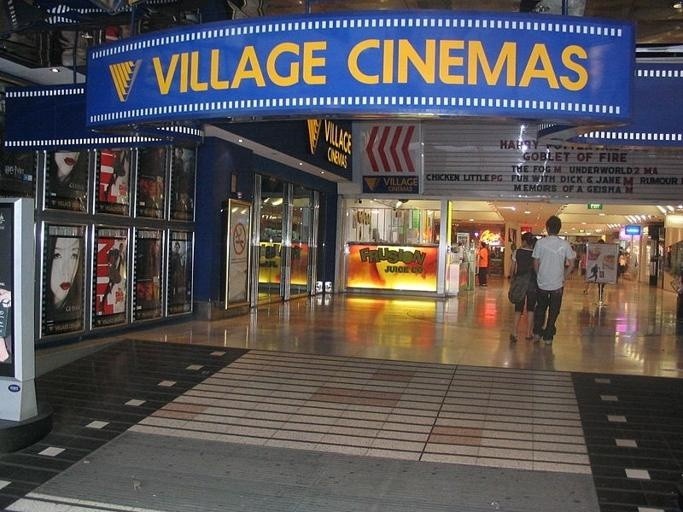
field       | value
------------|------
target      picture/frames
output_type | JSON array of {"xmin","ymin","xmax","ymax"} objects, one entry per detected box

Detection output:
[
  {"xmin": 133, "ymin": 145, "xmax": 169, "ymax": 220},
  {"xmin": 92, "ymin": 146, "xmax": 133, "ymax": 218},
  {"xmin": 37, "ymin": 220, "xmax": 87, "ymax": 341},
  {"xmin": 167, "ymin": 144, "xmax": 198, "ymax": 224},
  {"xmin": 41, "ymin": 149, "xmax": 90, "ymax": 215},
  {"xmin": 89, "ymin": 222, "xmax": 129, "ymax": 331},
  {"xmin": 164, "ymin": 228, "xmax": 194, "ymax": 318},
  {"xmin": 0, "ymin": 146, "xmax": 39, "ymax": 209},
  {"xmin": 131, "ymin": 226, "xmax": 164, "ymax": 325}
]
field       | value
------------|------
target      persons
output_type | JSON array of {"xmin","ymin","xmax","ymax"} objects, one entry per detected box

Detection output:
[
  {"xmin": 531, "ymin": 214, "xmax": 575, "ymax": 348},
  {"xmin": 565, "ymin": 235, "xmax": 626, "ymax": 296},
  {"xmin": 48, "ymin": 141, "xmax": 187, "ymax": 322},
  {"xmin": 476, "ymin": 240, "xmax": 489, "ymax": 288},
  {"xmin": 507, "ymin": 233, "xmax": 536, "ymax": 345}
]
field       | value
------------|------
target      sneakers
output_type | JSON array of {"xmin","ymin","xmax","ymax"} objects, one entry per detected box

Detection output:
[{"xmin": 509, "ymin": 334, "xmax": 552, "ymax": 345}]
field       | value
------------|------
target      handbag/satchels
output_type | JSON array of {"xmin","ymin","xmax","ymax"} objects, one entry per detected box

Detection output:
[{"xmin": 508, "ymin": 274, "xmax": 531, "ymax": 304}]
